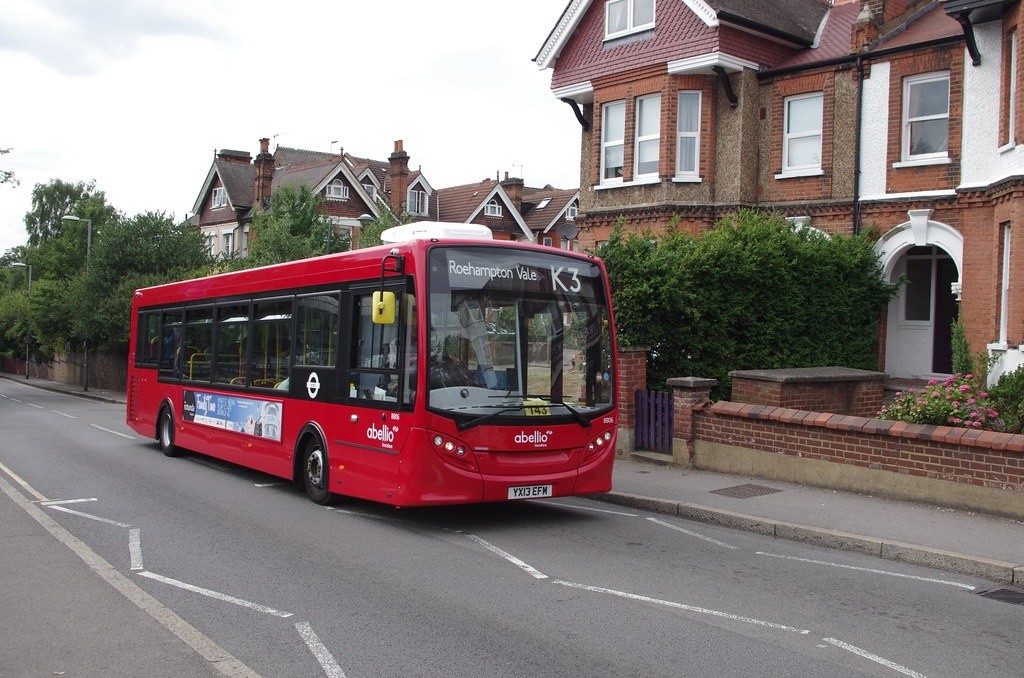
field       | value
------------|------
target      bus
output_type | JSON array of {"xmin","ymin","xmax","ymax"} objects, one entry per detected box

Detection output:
[{"xmin": 127, "ymin": 219, "xmax": 620, "ymax": 508}]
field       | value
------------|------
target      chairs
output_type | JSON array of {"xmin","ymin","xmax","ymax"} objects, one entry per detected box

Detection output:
[{"xmin": 150, "ymin": 336, "xmax": 283, "ymax": 389}]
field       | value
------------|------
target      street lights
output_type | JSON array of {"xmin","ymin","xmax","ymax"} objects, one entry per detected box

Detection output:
[
  {"xmin": 11, "ymin": 261, "xmax": 32, "ymax": 379},
  {"xmin": 62, "ymin": 215, "xmax": 91, "ymax": 391}
]
